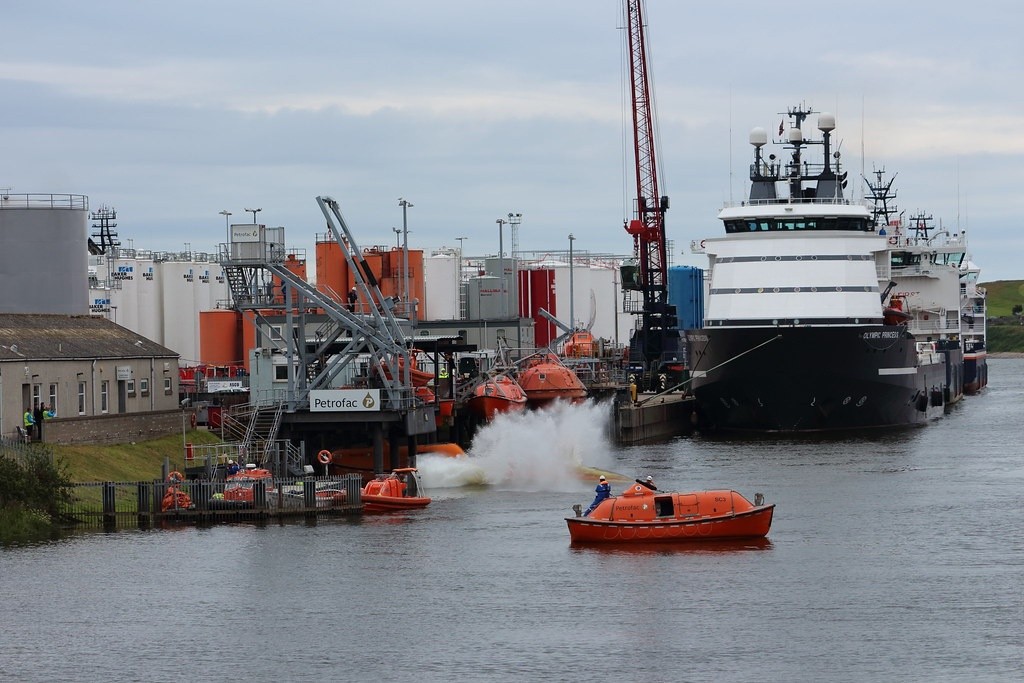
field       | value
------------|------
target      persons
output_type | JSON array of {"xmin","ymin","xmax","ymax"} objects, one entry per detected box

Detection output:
[
  {"xmin": 227, "ymin": 460, "xmax": 240, "ymax": 476},
  {"xmin": 879, "ymin": 223, "xmax": 886, "ymax": 235},
  {"xmin": 23, "ymin": 407, "xmax": 36, "ymax": 437},
  {"xmin": 583, "ymin": 475, "xmax": 612, "ymax": 517},
  {"xmin": 455, "ymin": 374, "xmax": 467, "ymax": 383},
  {"xmin": 439, "ymin": 367, "xmax": 449, "ymax": 378},
  {"xmin": 646, "ymin": 475, "xmax": 657, "ymax": 487},
  {"xmin": 347, "ymin": 286, "xmax": 359, "ymax": 315},
  {"xmin": 163, "ymin": 477, "xmax": 185, "ymax": 511},
  {"xmin": 34, "ymin": 402, "xmax": 57, "ymax": 440},
  {"xmin": 907, "ymin": 238, "xmax": 910, "ymax": 246},
  {"xmin": 895, "ymin": 226, "xmax": 901, "ymax": 247}
]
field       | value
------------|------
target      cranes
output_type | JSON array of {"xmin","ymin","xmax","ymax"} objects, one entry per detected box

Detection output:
[{"xmin": 616, "ymin": 0, "xmax": 688, "ymax": 402}]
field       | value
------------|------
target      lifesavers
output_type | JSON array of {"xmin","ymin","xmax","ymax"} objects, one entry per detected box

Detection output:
[
  {"xmin": 166, "ymin": 472, "xmax": 183, "ymax": 488},
  {"xmin": 889, "ymin": 236, "xmax": 899, "ymax": 244},
  {"xmin": 191, "ymin": 413, "xmax": 196, "ymax": 427},
  {"xmin": 318, "ymin": 450, "xmax": 332, "ymax": 463}
]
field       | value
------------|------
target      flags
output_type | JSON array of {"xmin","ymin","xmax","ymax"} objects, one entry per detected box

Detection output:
[{"xmin": 779, "ymin": 121, "xmax": 784, "ymax": 136}]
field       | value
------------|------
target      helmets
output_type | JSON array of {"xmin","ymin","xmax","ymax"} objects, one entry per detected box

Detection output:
[
  {"xmin": 228, "ymin": 460, "xmax": 233, "ymax": 464},
  {"xmin": 647, "ymin": 476, "xmax": 652, "ymax": 480},
  {"xmin": 442, "ymin": 368, "xmax": 445, "ymax": 371},
  {"xmin": 352, "ymin": 287, "xmax": 357, "ymax": 291},
  {"xmin": 599, "ymin": 475, "xmax": 605, "ymax": 479}
]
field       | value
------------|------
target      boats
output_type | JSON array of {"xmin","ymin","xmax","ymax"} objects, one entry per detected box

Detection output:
[
  {"xmin": 328, "ymin": 441, "xmax": 470, "ymax": 488},
  {"xmin": 884, "ymin": 308, "xmax": 913, "ymax": 327},
  {"xmin": 208, "ymin": 463, "xmax": 275, "ymax": 510},
  {"xmin": 175, "ymin": 194, "xmax": 709, "ymax": 519},
  {"xmin": 681, "ymin": 94, "xmax": 991, "ymax": 441},
  {"xmin": 517, "ymin": 363, "xmax": 589, "ymax": 409},
  {"xmin": 467, "ymin": 373, "xmax": 529, "ymax": 424},
  {"xmin": 563, "ymin": 478, "xmax": 777, "ymax": 545},
  {"xmin": 360, "ymin": 467, "xmax": 432, "ymax": 515},
  {"xmin": 561, "ymin": 330, "xmax": 598, "ymax": 358},
  {"xmin": 370, "ymin": 353, "xmax": 436, "ymax": 387}
]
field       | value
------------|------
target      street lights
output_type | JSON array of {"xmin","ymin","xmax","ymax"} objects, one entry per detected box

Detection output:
[
  {"xmin": 218, "ymin": 209, "xmax": 232, "ymax": 309},
  {"xmin": 496, "ymin": 218, "xmax": 508, "ymax": 319},
  {"xmin": 392, "ymin": 226, "xmax": 402, "ymax": 318},
  {"xmin": 568, "ymin": 232, "xmax": 577, "ymax": 329},
  {"xmin": 245, "ymin": 208, "xmax": 263, "ymax": 304},
  {"xmin": 397, "ymin": 196, "xmax": 414, "ymax": 320}
]
